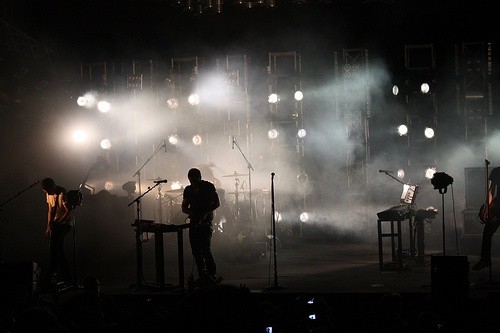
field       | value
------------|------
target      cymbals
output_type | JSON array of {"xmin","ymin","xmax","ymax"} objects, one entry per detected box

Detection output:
[
  {"xmin": 226, "ymin": 190, "xmax": 245, "ymax": 194},
  {"xmin": 223, "ymin": 174, "xmax": 248, "ymax": 177},
  {"xmin": 166, "ymin": 186, "xmax": 184, "ymax": 193}
]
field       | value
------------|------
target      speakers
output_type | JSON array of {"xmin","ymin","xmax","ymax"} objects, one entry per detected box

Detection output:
[{"xmin": 430, "ymin": 256, "xmax": 470, "ymax": 297}]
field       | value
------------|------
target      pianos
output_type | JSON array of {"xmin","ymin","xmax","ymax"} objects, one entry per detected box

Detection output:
[
  {"xmin": 131, "ymin": 218, "xmax": 183, "ymax": 233},
  {"xmin": 377, "ymin": 204, "xmax": 416, "ymax": 221}
]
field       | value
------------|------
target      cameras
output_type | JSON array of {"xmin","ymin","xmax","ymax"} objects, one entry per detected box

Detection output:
[
  {"xmin": 307, "ymin": 312, "xmax": 317, "ymax": 320},
  {"xmin": 264, "ymin": 326, "xmax": 273, "ymax": 333},
  {"xmin": 303, "ymin": 296, "xmax": 315, "ymax": 305}
]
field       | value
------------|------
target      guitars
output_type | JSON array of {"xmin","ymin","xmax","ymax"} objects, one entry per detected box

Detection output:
[
  {"xmin": 478, "ymin": 196, "xmax": 500, "ymax": 224},
  {"xmin": 44, "ymin": 217, "xmax": 62, "ymax": 241}
]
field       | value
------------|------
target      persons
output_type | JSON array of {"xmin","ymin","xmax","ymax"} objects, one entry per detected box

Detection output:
[
  {"xmin": 182, "ymin": 167, "xmax": 220, "ymax": 279},
  {"xmin": 472, "ymin": 166, "xmax": 500, "ymax": 269},
  {"xmin": 42, "ymin": 177, "xmax": 75, "ymax": 238}
]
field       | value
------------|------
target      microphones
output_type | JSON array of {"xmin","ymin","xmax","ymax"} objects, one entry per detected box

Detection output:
[
  {"xmin": 379, "ymin": 170, "xmax": 393, "ymax": 172},
  {"xmin": 485, "ymin": 159, "xmax": 490, "ymax": 164},
  {"xmin": 154, "ymin": 180, "xmax": 167, "ymax": 183},
  {"xmin": 232, "ymin": 136, "xmax": 234, "ymax": 148},
  {"xmin": 164, "ymin": 139, "xmax": 167, "ymax": 151}
]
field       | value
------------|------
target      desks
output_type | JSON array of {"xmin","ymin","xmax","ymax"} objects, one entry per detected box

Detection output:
[
  {"xmin": 378, "ymin": 216, "xmax": 413, "ymax": 271},
  {"xmin": 136, "ymin": 226, "xmax": 185, "ymax": 289}
]
